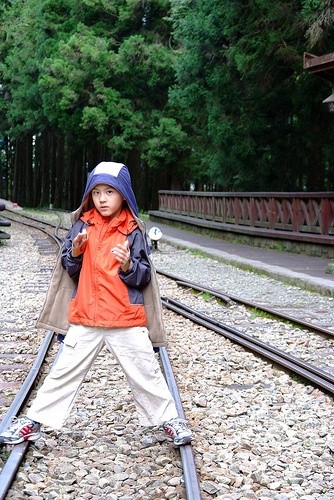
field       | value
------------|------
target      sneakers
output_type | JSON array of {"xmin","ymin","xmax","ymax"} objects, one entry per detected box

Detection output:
[
  {"xmin": 164, "ymin": 417, "xmax": 196, "ymax": 445},
  {"xmin": 0, "ymin": 415, "xmax": 42, "ymax": 444}
]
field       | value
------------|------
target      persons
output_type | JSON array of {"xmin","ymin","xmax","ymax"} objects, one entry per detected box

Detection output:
[{"xmin": 0, "ymin": 161, "xmax": 197, "ymax": 447}]
